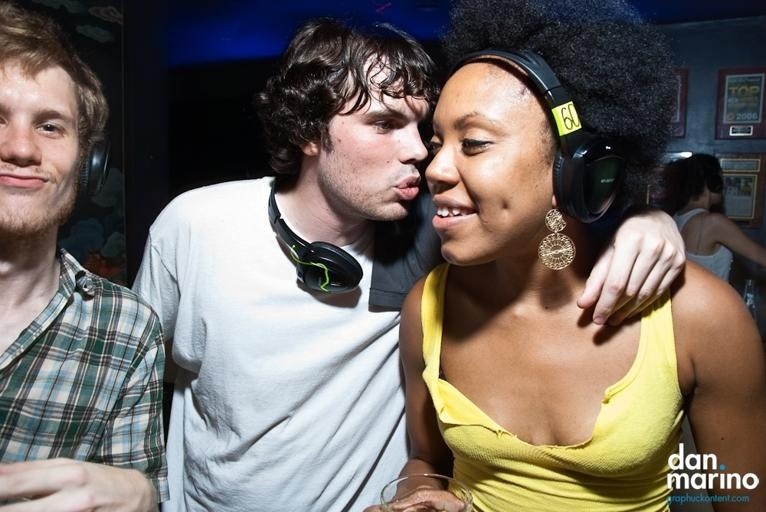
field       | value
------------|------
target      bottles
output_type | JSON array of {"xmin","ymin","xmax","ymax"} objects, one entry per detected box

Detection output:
[{"xmin": 742, "ymin": 278, "xmax": 758, "ymax": 326}]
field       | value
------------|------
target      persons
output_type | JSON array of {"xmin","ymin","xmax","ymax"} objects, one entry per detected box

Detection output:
[
  {"xmin": 656, "ymin": 151, "xmax": 766, "ymax": 286},
  {"xmin": 0, "ymin": 0, "xmax": 171, "ymax": 511},
  {"xmin": 126, "ymin": 12, "xmax": 687, "ymax": 510},
  {"xmin": 367, "ymin": 1, "xmax": 766, "ymax": 511}
]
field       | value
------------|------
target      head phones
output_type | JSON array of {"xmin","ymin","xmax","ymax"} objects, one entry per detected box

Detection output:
[
  {"xmin": 80, "ymin": 136, "xmax": 113, "ymax": 201},
  {"xmin": 443, "ymin": 41, "xmax": 625, "ymax": 228},
  {"xmin": 265, "ymin": 178, "xmax": 363, "ymax": 296}
]
